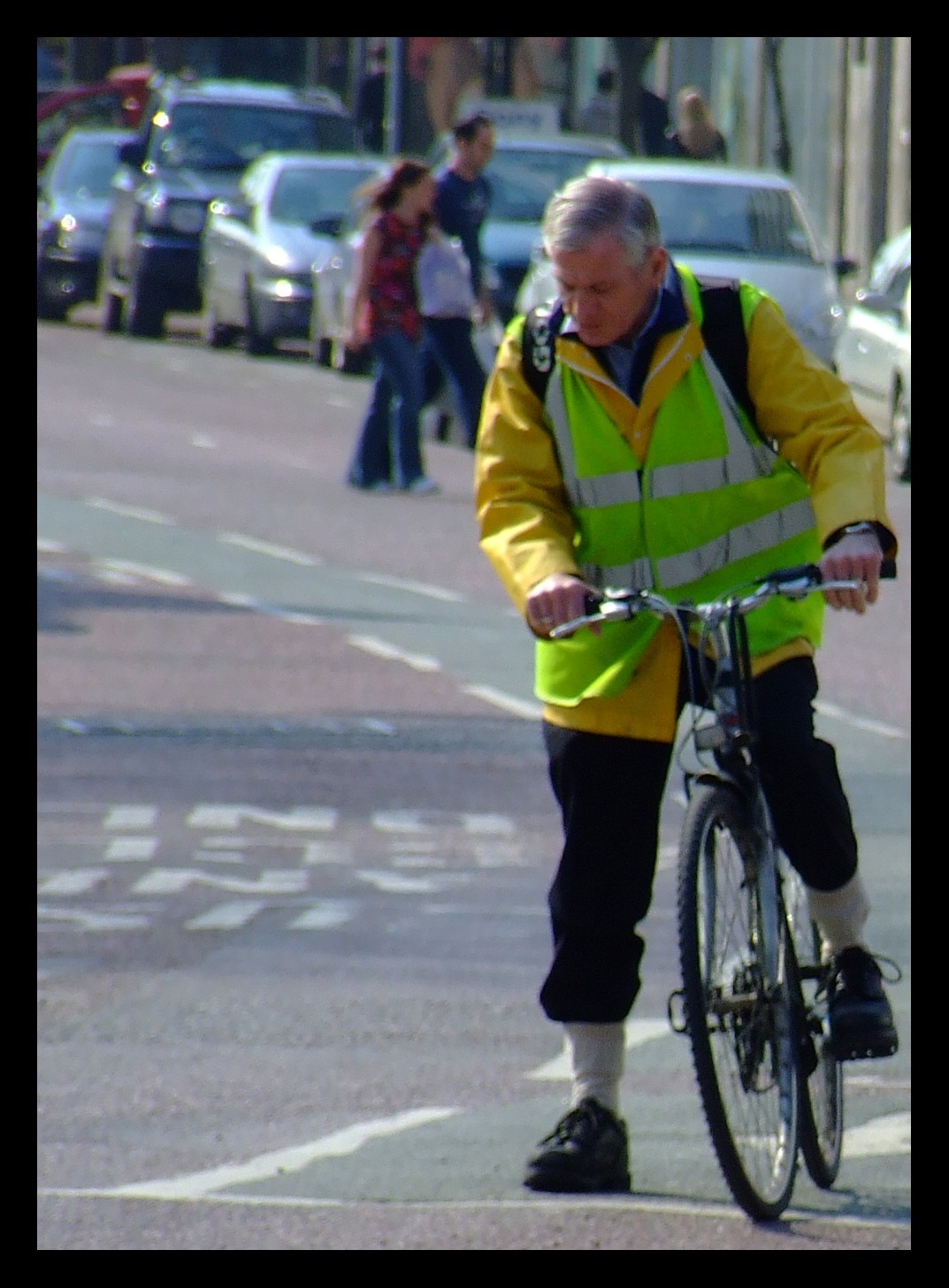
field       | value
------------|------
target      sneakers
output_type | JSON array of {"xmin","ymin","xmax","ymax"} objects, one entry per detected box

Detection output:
[
  {"xmin": 814, "ymin": 945, "xmax": 903, "ymax": 1061},
  {"xmin": 523, "ymin": 1096, "xmax": 632, "ymax": 1193}
]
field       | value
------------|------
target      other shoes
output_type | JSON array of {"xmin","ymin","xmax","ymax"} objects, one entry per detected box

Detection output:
[
  {"xmin": 402, "ymin": 475, "xmax": 442, "ymax": 495},
  {"xmin": 349, "ymin": 472, "xmax": 396, "ymax": 493}
]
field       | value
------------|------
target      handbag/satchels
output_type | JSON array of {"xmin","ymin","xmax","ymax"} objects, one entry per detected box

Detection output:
[{"xmin": 416, "ymin": 228, "xmax": 476, "ymax": 319}]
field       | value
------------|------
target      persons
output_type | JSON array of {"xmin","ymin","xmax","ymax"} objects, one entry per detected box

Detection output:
[
  {"xmin": 351, "ymin": 42, "xmax": 409, "ymax": 153},
  {"xmin": 348, "ymin": 116, "xmax": 497, "ymax": 491},
  {"xmin": 474, "ymin": 179, "xmax": 900, "ymax": 1190},
  {"xmin": 583, "ymin": 70, "xmax": 727, "ymax": 160}
]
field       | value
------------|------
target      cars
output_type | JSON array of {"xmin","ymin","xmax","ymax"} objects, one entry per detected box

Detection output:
[
  {"xmin": 518, "ymin": 159, "xmax": 847, "ymax": 404},
  {"xmin": 315, "ymin": 125, "xmax": 638, "ymax": 388},
  {"xmin": 832, "ymin": 230, "xmax": 910, "ymax": 479},
  {"xmin": 34, "ymin": 82, "xmax": 337, "ymax": 340},
  {"xmin": 202, "ymin": 148, "xmax": 411, "ymax": 353}
]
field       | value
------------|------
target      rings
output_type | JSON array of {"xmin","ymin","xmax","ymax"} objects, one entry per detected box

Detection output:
[{"xmin": 541, "ymin": 614, "xmax": 554, "ymax": 624}]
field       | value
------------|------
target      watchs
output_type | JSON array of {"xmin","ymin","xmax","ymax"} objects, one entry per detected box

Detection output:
[{"xmin": 840, "ymin": 521, "xmax": 875, "ymax": 535}]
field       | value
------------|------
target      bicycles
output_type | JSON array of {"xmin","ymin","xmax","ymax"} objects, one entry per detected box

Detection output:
[{"xmin": 525, "ymin": 567, "xmax": 874, "ymax": 1225}]
